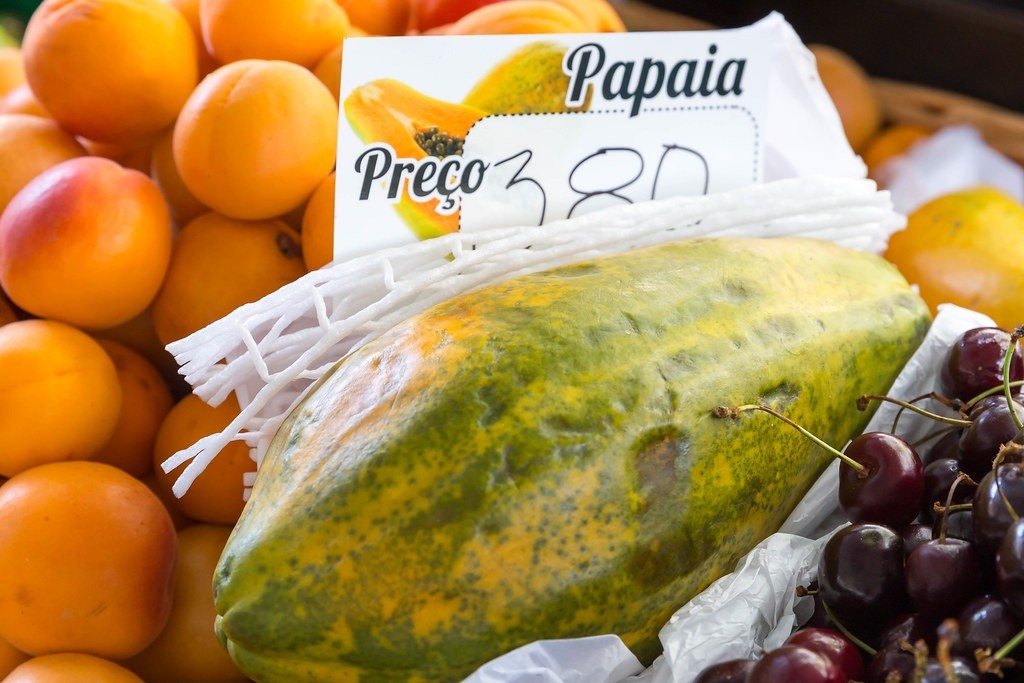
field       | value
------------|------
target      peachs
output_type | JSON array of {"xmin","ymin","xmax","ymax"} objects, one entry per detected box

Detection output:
[{"xmin": 0, "ymin": 1, "xmax": 929, "ymax": 683}]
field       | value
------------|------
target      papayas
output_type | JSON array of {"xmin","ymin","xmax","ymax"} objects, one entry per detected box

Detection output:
[{"xmin": 214, "ymin": 234, "xmax": 936, "ymax": 683}]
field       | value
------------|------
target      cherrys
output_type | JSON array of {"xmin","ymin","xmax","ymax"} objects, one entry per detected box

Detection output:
[{"xmin": 692, "ymin": 327, "xmax": 1024, "ymax": 683}]
